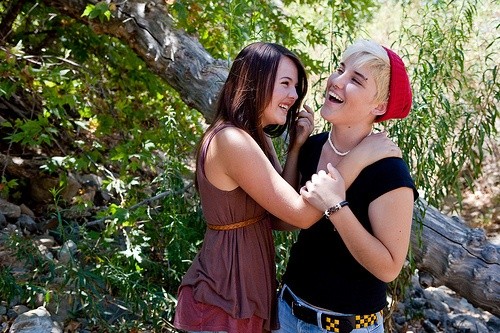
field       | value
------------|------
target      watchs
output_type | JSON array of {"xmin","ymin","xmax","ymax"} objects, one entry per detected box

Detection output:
[{"xmin": 322, "ymin": 201, "xmax": 348, "ymax": 219}]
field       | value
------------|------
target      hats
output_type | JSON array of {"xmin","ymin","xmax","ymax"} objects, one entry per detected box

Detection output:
[{"xmin": 373, "ymin": 46, "xmax": 412, "ymax": 121}]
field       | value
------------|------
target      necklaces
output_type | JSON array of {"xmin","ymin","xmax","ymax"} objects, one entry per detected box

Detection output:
[{"xmin": 328, "ymin": 125, "xmax": 372, "ymax": 157}]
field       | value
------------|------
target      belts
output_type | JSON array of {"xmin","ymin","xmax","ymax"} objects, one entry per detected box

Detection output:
[{"xmin": 279, "ymin": 287, "xmax": 384, "ymax": 333}]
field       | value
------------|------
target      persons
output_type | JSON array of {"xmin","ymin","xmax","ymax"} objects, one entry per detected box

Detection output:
[
  {"xmin": 173, "ymin": 42, "xmax": 403, "ymax": 333},
  {"xmin": 277, "ymin": 39, "xmax": 419, "ymax": 333}
]
